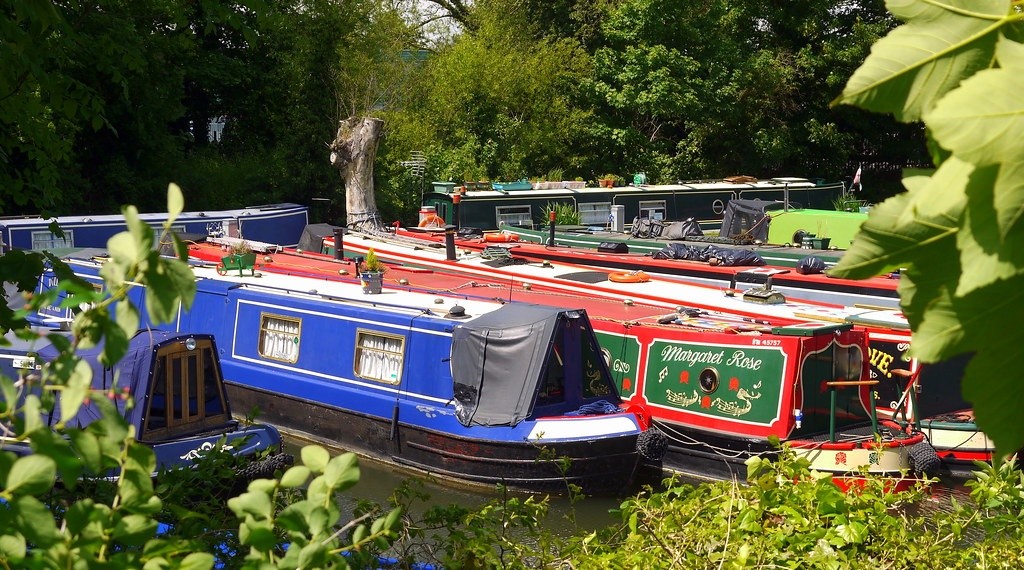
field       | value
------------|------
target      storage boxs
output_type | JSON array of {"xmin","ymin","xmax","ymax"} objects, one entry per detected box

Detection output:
[{"xmin": 494, "ymin": 181, "xmax": 586, "ymax": 191}]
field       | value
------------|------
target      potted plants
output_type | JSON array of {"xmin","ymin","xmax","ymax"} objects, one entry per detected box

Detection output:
[
  {"xmin": 360, "ymin": 248, "xmax": 386, "ymax": 294},
  {"xmin": 463, "ymin": 173, "xmax": 476, "ymax": 191},
  {"xmin": 222, "ymin": 236, "xmax": 256, "ymax": 271},
  {"xmin": 477, "ymin": 175, "xmax": 491, "ymax": 190},
  {"xmin": 597, "ymin": 172, "xmax": 626, "ymax": 188}
]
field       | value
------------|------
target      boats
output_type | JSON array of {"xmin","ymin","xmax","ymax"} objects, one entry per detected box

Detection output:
[
  {"xmin": 0, "ymin": 203, "xmax": 310, "ymax": 268},
  {"xmin": 186, "ymin": 174, "xmax": 1021, "ymax": 502},
  {"xmin": 27, "ymin": 247, "xmax": 644, "ymax": 503},
  {"xmin": 0, "ymin": 316, "xmax": 284, "ymax": 501}
]
